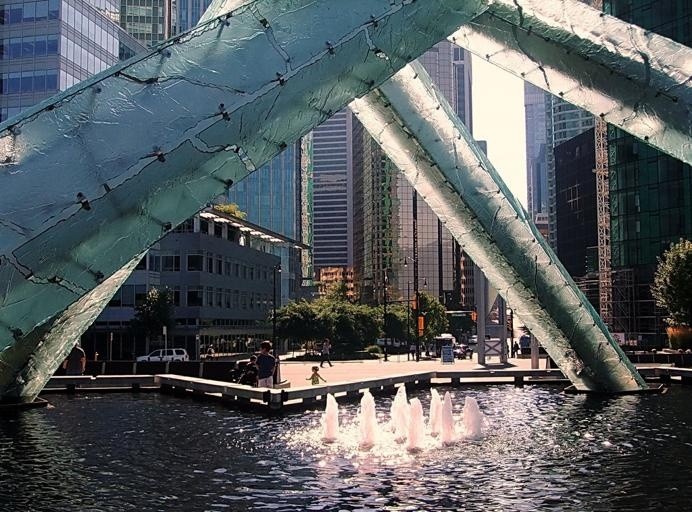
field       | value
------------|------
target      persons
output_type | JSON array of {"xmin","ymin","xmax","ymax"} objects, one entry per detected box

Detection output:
[
  {"xmin": 62, "ymin": 338, "xmax": 87, "ymax": 388},
  {"xmin": 513, "ymin": 341, "xmax": 521, "ymax": 358},
  {"xmin": 410, "ymin": 343, "xmax": 416, "ymax": 361},
  {"xmin": 206, "ymin": 345, "xmax": 215, "ymax": 360},
  {"xmin": 238, "ymin": 340, "xmax": 276, "ymax": 402},
  {"xmin": 320, "ymin": 338, "xmax": 332, "ymax": 368},
  {"xmin": 306, "ymin": 366, "xmax": 326, "ymax": 385}
]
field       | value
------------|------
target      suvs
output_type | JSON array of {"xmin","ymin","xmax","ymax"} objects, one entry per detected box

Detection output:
[{"xmin": 136, "ymin": 347, "xmax": 190, "ymax": 362}]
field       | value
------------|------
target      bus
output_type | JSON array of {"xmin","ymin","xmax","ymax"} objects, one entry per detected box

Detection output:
[{"xmin": 433, "ymin": 333, "xmax": 456, "ymax": 357}]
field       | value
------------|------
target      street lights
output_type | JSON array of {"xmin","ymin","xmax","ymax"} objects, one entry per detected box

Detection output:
[
  {"xmin": 506, "ymin": 306, "xmax": 518, "ymax": 358},
  {"xmin": 381, "ymin": 266, "xmax": 392, "ymax": 360},
  {"xmin": 406, "ymin": 276, "xmax": 427, "ymax": 360},
  {"xmin": 398, "ymin": 255, "xmax": 420, "ymax": 361},
  {"xmin": 272, "ymin": 262, "xmax": 290, "ymax": 390}
]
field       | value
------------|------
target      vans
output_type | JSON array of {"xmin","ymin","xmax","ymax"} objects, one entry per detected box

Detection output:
[{"xmin": 376, "ymin": 337, "xmax": 407, "ymax": 349}]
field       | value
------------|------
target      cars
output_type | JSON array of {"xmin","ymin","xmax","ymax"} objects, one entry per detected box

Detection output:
[
  {"xmin": 451, "ymin": 344, "xmax": 475, "ymax": 360},
  {"xmin": 519, "ymin": 334, "xmax": 531, "ymax": 344},
  {"xmin": 466, "ymin": 334, "xmax": 479, "ymax": 344}
]
